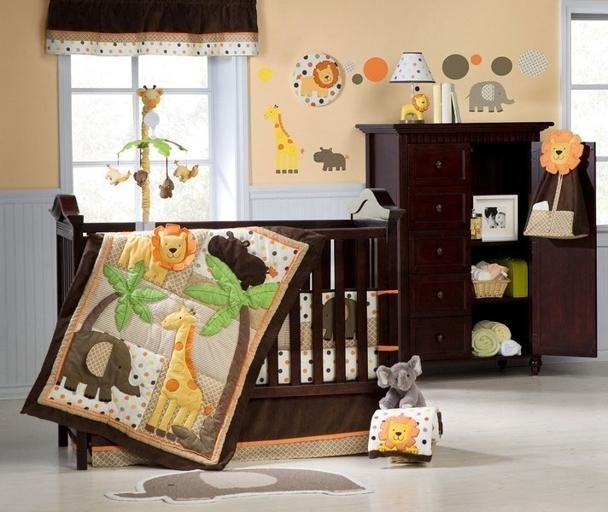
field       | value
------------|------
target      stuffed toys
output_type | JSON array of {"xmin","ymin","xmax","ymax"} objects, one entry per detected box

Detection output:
[
  {"xmin": 375, "ymin": 357, "xmax": 426, "ymax": 408},
  {"xmin": 105, "ymin": 158, "xmax": 197, "ymax": 198}
]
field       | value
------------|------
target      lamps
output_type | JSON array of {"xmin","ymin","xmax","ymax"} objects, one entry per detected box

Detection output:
[{"xmin": 388, "ymin": 50, "xmax": 435, "ymax": 124}]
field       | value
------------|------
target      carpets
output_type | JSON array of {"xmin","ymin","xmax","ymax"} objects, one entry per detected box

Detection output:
[{"xmin": 103, "ymin": 462, "xmax": 377, "ymax": 506}]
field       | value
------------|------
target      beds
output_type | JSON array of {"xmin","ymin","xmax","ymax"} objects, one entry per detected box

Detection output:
[{"xmin": 44, "ymin": 186, "xmax": 408, "ymax": 470}]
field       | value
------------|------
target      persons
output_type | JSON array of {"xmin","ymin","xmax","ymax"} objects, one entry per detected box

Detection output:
[
  {"xmin": 494, "ymin": 212, "xmax": 506, "ymax": 228},
  {"xmin": 485, "ymin": 206, "xmax": 498, "ymax": 229}
]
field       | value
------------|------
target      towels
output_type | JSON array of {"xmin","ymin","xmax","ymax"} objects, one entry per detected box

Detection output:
[
  {"xmin": 474, "ymin": 319, "xmax": 512, "ymax": 342},
  {"xmin": 471, "ymin": 328, "xmax": 500, "ymax": 358},
  {"xmin": 499, "ymin": 339, "xmax": 522, "ymax": 357}
]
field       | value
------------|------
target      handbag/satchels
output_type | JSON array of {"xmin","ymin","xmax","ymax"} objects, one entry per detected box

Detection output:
[{"xmin": 520, "ymin": 164, "xmax": 590, "ymax": 240}]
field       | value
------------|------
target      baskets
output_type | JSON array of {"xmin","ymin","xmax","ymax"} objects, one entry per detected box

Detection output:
[{"xmin": 472, "ymin": 274, "xmax": 511, "ymax": 298}]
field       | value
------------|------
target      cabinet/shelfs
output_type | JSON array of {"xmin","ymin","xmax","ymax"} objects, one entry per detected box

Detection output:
[{"xmin": 353, "ymin": 119, "xmax": 598, "ymax": 375}]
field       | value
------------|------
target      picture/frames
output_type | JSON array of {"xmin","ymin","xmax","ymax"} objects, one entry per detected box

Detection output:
[{"xmin": 471, "ymin": 193, "xmax": 520, "ymax": 243}]
